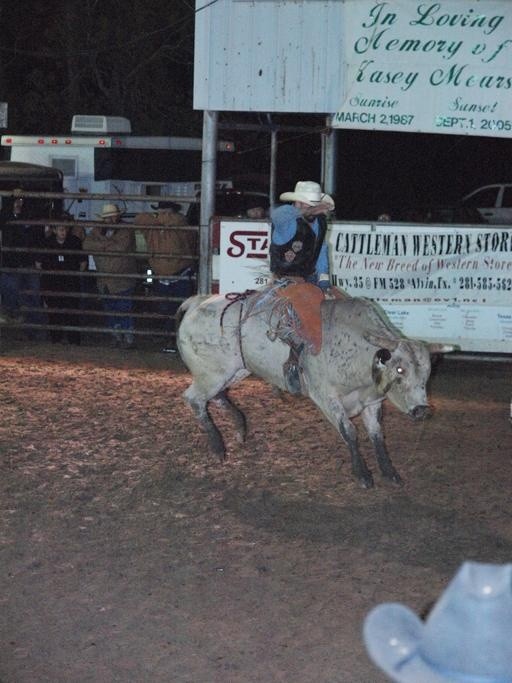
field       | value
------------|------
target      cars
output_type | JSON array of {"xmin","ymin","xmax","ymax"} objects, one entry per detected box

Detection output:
[{"xmin": 461, "ymin": 184, "xmax": 512, "ymax": 224}]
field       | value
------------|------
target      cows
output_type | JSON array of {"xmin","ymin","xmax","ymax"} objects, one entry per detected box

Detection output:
[{"xmin": 174, "ymin": 284, "xmax": 460, "ymax": 490}]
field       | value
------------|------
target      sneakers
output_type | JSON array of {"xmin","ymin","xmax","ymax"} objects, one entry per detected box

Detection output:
[
  {"xmin": 284, "ymin": 362, "xmax": 301, "ymax": 395},
  {"xmin": 109, "ymin": 334, "xmax": 137, "ymax": 348}
]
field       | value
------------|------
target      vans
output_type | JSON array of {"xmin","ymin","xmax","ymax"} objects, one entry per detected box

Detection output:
[{"xmin": 188, "ymin": 188, "xmax": 270, "ymax": 222}]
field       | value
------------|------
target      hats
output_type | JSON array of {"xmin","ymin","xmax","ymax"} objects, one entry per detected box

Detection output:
[
  {"xmin": 243, "ymin": 202, "xmax": 266, "ymax": 211},
  {"xmin": 281, "ymin": 181, "xmax": 335, "ymax": 210},
  {"xmin": 93, "ymin": 203, "xmax": 127, "ymax": 217},
  {"xmin": 150, "ymin": 200, "xmax": 181, "ymax": 211}
]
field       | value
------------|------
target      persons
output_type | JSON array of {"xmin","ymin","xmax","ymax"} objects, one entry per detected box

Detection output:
[
  {"xmin": 135, "ymin": 200, "xmax": 197, "ymax": 353},
  {"xmin": 83, "ymin": 205, "xmax": 138, "ymax": 349},
  {"xmin": 35, "ymin": 226, "xmax": 88, "ymax": 345},
  {"xmin": 269, "ymin": 180, "xmax": 335, "ymax": 395},
  {"xmin": 0, "ymin": 197, "xmax": 48, "ymax": 342}
]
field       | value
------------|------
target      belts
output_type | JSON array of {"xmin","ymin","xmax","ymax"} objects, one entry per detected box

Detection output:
[{"xmin": 159, "ymin": 268, "xmax": 191, "ymax": 285}]
